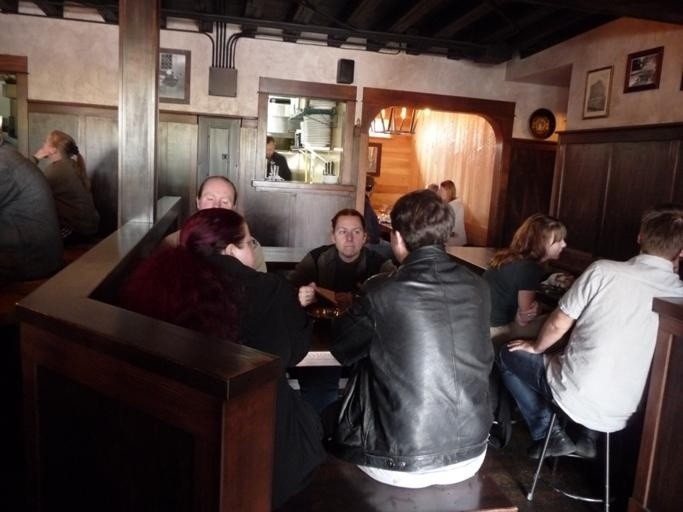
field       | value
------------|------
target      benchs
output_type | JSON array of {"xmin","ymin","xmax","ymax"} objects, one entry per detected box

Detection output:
[
  {"xmin": 272, "ymin": 462, "xmax": 519, "ymax": 512},
  {"xmin": 0, "ymin": 230, "xmax": 112, "ymax": 327}
]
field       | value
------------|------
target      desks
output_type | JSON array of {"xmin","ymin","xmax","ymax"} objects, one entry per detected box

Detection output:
[
  {"xmin": 445, "ymin": 245, "xmax": 509, "ymax": 270},
  {"xmin": 379, "ymin": 219, "xmax": 391, "ymax": 232},
  {"xmin": 262, "ymin": 246, "xmax": 306, "ymax": 262},
  {"xmin": 629, "ymin": 296, "xmax": 683, "ymax": 511},
  {"xmin": 294, "ymin": 350, "xmax": 342, "ymax": 367}
]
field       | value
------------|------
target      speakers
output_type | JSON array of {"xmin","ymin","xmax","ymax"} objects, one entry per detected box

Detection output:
[{"xmin": 337, "ymin": 58, "xmax": 354, "ymax": 84}]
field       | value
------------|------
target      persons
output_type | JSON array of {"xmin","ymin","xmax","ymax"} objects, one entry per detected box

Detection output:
[
  {"xmin": 363, "ymin": 174, "xmax": 391, "ymax": 245},
  {"xmin": 161, "ymin": 174, "xmax": 268, "ymax": 275},
  {"xmin": 265, "ymin": 135, "xmax": 291, "ymax": 181},
  {"xmin": 479, "ymin": 212, "xmax": 571, "ymax": 344},
  {"xmin": 24, "ymin": 131, "xmax": 102, "ymax": 247},
  {"xmin": 285, "ymin": 207, "xmax": 387, "ymax": 307},
  {"xmin": 438, "ymin": 179, "xmax": 467, "ymax": 247},
  {"xmin": 495, "ymin": 202, "xmax": 682, "ymax": 462},
  {"xmin": 304, "ymin": 188, "xmax": 495, "ymax": 490},
  {"xmin": 0, "ymin": 130, "xmax": 63, "ymax": 282},
  {"xmin": 123, "ymin": 207, "xmax": 325, "ymax": 512}
]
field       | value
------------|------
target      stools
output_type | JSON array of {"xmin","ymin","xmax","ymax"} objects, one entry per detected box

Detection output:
[{"xmin": 526, "ymin": 398, "xmax": 644, "ymax": 512}]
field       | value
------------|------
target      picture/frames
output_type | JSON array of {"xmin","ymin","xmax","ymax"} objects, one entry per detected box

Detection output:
[
  {"xmin": 623, "ymin": 45, "xmax": 664, "ymax": 93},
  {"xmin": 528, "ymin": 108, "xmax": 556, "ymax": 139},
  {"xmin": 159, "ymin": 48, "xmax": 191, "ymax": 105},
  {"xmin": 367, "ymin": 142, "xmax": 382, "ymax": 176},
  {"xmin": 583, "ymin": 64, "xmax": 613, "ymax": 120}
]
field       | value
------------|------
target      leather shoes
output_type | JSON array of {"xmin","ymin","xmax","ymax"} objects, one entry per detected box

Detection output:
[
  {"xmin": 575, "ymin": 435, "xmax": 597, "ymax": 458},
  {"xmin": 526, "ymin": 428, "xmax": 577, "ymax": 459}
]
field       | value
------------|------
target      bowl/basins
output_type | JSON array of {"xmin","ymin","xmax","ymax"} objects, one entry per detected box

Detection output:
[
  {"xmin": 322, "ymin": 176, "xmax": 339, "ymax": 184},
  {"xmin": 306, "ymin": 307, "xmax": 346, "ymax": 331}
]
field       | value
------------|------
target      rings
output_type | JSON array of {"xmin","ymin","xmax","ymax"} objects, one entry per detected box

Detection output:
[{"xmin": 527, "ymin": 313, "xmax": 530, "ymax": 317}]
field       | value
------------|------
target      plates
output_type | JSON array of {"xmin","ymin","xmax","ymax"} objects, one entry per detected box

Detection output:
[
  {"xmin": 290, "ymin": 98, "xmax": 336, "ymax": 114},
  {"xmin": 294, "ymin": 114, "xmax": 332, "ymax": 149}
]
